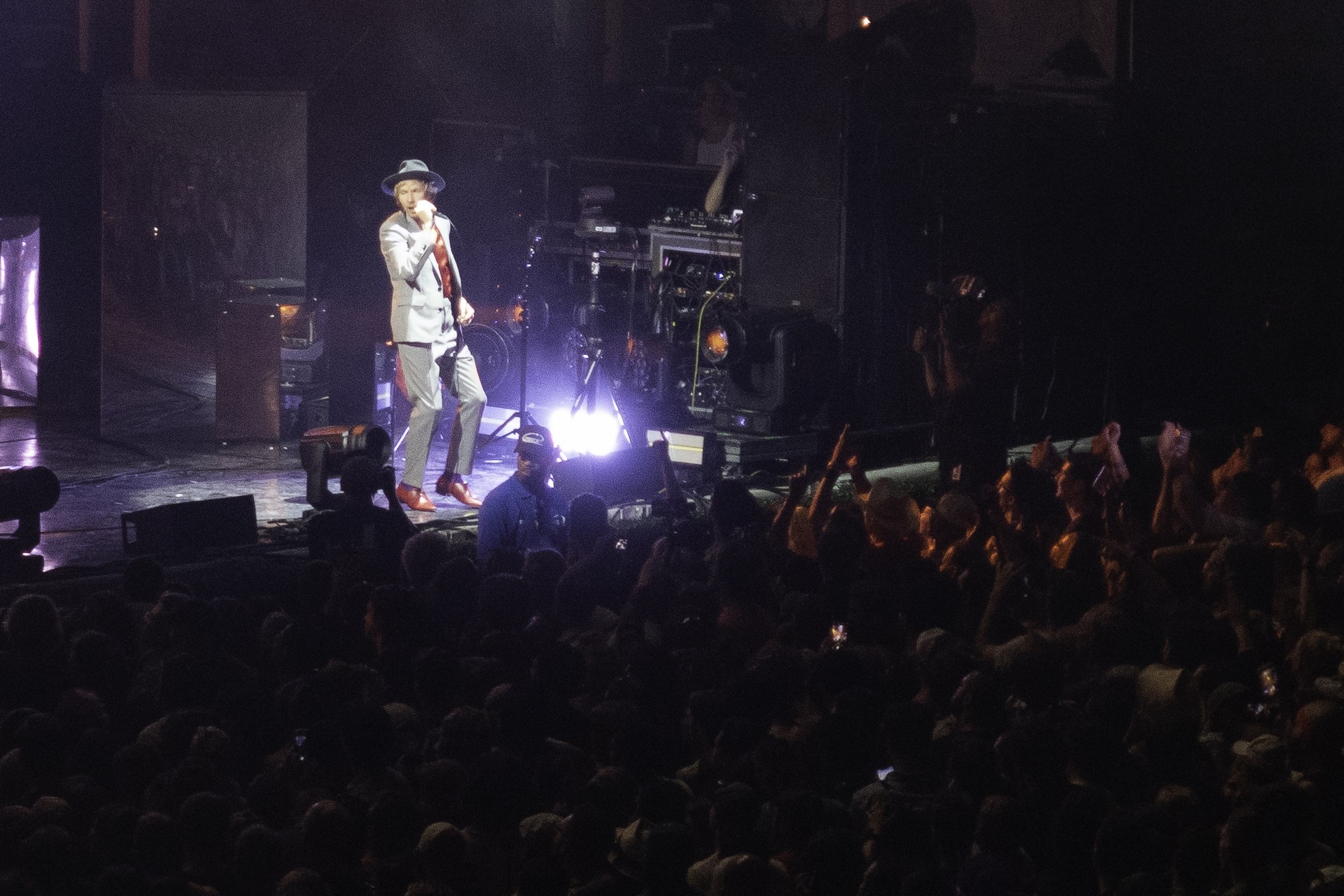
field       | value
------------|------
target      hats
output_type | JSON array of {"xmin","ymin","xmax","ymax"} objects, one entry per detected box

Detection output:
[
  {"xmin": 855, "ymin": 478, "xmax": 919, "ymax": 533},
  {"xmin": 513, "ymin": 425, "xmax": 553, "ymax": 456},
  {"xmin": 382, "ymin": 159, "xmax": 446, "ymax": 197}
]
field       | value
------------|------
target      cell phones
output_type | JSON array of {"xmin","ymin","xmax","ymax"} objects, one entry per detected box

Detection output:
[{"xmin": 829, "ymin": 620, "xmax": 848, "ymax": 652}]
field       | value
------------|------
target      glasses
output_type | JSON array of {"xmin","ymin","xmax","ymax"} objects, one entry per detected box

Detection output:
[
  {"xmin": 1058, "ymin": 469, "xmax": 1072, "ymax": 483},
  {"xmin": 994, "ymin": 478, "xmax": 1013, "ymax": 497}
]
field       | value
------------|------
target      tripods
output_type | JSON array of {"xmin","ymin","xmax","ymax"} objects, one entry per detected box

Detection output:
[{"xmin": 474, "ymin": 242, "xmax": 568, "ymax": 462}]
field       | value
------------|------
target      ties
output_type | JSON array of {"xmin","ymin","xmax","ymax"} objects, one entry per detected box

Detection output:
[{"xmin": 536, "ymin": 492, "xmax": 551, "ymax": 538}]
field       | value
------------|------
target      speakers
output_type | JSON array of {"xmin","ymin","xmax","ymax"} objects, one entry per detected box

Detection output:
[
  {"xmin": 737, "ymin": 60, "xmax": 879, "ymax": 343},
  {"xmin": 551, "ymin": 439, "xmax": 687, "ymax": 508},
  {"xmin": 119, "ymin": 493, "xmax": 258, "ymax": 557}
]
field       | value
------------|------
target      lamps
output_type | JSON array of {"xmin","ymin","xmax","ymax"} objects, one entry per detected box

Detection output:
[
  {"xmin": 0, "ymin": 464, "xmax": 57, "ymax": 558},
  {"xmin": 299, "ymin": 424, "xmax": 392, "ymax": 513}
]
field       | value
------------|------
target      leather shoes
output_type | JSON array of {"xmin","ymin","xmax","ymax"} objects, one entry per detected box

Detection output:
[
  {"xmin": 396, "ymin": 479, "xmax": 436, "ymax": 512},
  {"xmin": 436, "ymin": 473, "xmax": 483, "ymax": 508}
]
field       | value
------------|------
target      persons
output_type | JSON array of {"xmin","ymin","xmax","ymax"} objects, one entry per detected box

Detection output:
[
  {"xmin": 694, "ymin": 74, "xmax": 748, "ymax": 217},
  {"xmin": 912, "ymin": 271, "xmax": 1024, "ymax": 512},
  {"xmin": 378, "ymin": 158, "xmax": 488, "ymax": 515},
  {"xmin": 0, "ymin": 409, "xmax": 1344, "ymax": 896}
]
